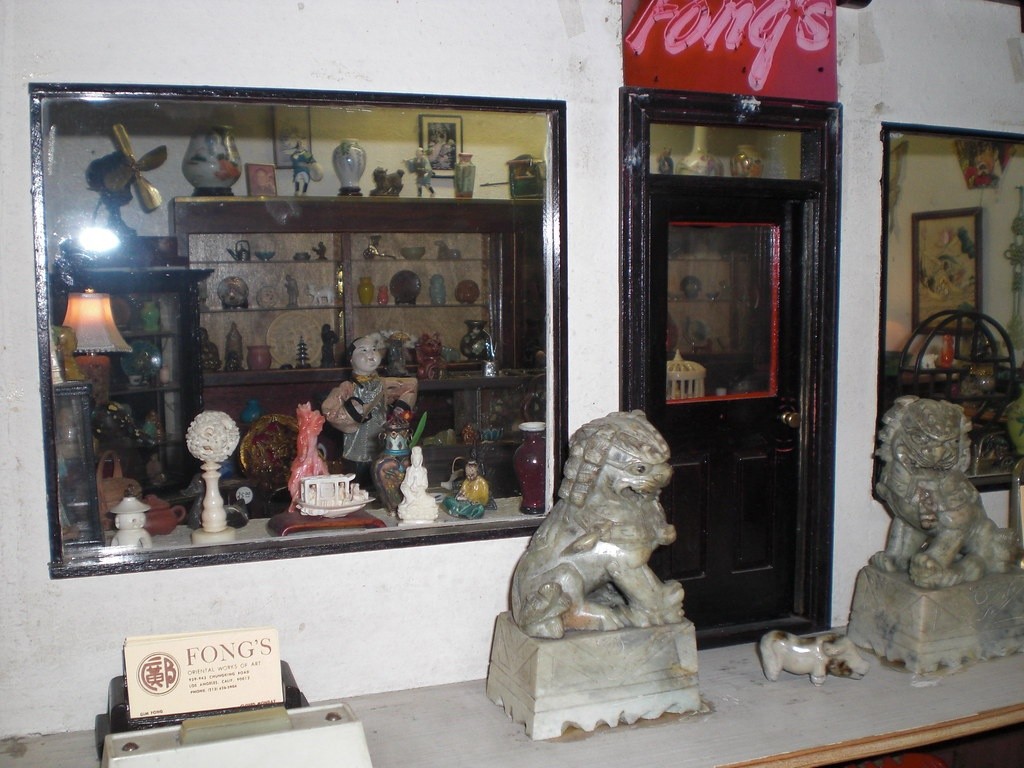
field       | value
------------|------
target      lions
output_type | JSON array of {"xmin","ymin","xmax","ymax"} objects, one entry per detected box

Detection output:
[
  {"xmin": 511, "ymin": 409, "xmax": 684, "ymax": 639},
  {"xmin": 875, "ymin": 394, "xmax": 1011, "ymax": 588}
]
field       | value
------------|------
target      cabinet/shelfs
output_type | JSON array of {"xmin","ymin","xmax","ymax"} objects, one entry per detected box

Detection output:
[
  {"xmin": 172, "ymin": 197, "xmax": 543, "ymax": 476},
  {"xmin": 671, "ymin": 223, "xmax": 763, "ymax": 366}
]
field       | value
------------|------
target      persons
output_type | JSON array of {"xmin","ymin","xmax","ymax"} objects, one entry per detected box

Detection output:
[
  {"xmin": 291, "ymin": 140, "xmax": 320, "ymax": 197},
  {"xmin": 252, "ymin": 167, "xmax": 274, "ymax": 194},
  {"xmin": 397, "ymin": 446, "xmax": 436, "ymax": 505},
  {"xmin": 283, "ymin": 275, "xmax": 300, "ymax": 306},
  {"xmin": 324, "ymin": 335, "xmax": 418, "ymax": 490},
  {"xmin": 402, "ymin": 147, "xmax": 437, "ymax": 198},
  {"xmin": 443, "ymin": 462, "xmax": 489, "ymax": 520}
]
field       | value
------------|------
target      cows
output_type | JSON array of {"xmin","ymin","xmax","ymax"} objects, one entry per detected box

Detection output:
[{"xmin": 760, "ymin": 630, "xmax": 869, "ymax": 687}]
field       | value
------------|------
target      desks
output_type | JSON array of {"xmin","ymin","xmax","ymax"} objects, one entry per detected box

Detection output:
[{"xmin": 1, "ymin": 627, "xmax": 1024, "ymax": 768}]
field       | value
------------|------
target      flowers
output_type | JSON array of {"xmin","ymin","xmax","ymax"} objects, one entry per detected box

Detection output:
[{"xmin": 952, "ymin": 132, "xmax": 1016, "ymax": 191}]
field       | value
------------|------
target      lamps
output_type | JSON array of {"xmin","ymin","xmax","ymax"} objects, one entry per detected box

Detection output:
[{"xmin": 63, "ymin": 293, "xmax": 133, "ymax": 358}]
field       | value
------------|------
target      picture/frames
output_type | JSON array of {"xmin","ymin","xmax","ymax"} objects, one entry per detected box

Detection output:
[
  {"xmin": 247, "ymin": 162, "xmax": 279, "ymax": 200},
  {"xmin": 273, "ymin": 102, "xmax": 315, "ymax": 170},
  {"xmin": 421, "ymin": 115, "xmax": 465, "ymax": 177},
  {"xmin": 908, "ymin": 208, "xmax": 984, "ymax": 336}
]
